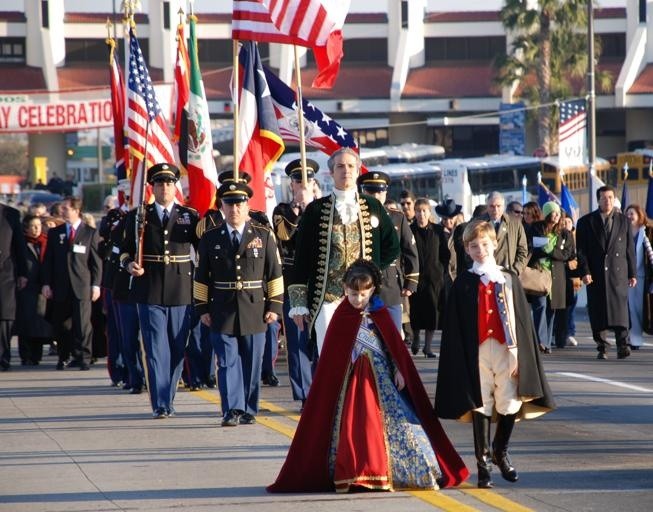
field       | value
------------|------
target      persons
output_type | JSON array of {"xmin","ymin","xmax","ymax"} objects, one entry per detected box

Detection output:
[
  {"xmin": 576, "ymin": 186, "xmax": 637, "ymax": 358},
  {"xmin": 433, "ymin": 220, "xmax": 556, "ymax": 489},
  {"xmin": 626, "ymin": 204, "xmax": 653, "ymax": 349},
  {"xmin": 263, "ymin": 258, "xmax": 473, "ymax": 492},
  {"xmin": 193, "ymin": 182, "xmax": 288, "ymax": 426},
  {"xmin": 119, "ymin": 161, "xmax": 200, "ymax": 419},
  {"xmin": 0, "ymin": 145, "xmax": 583, "ymax": 395}
]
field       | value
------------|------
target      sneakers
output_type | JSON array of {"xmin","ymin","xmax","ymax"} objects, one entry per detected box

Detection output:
[{"xmin": 540, "ymin": 336, "xmax": 578, "ymax": 353}]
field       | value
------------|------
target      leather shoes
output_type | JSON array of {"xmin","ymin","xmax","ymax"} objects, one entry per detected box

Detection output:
[
  {"xmin": 597, "ymin": 347, "xmax": 630, "ymax": 359},
  {"xmin": 111, "ymin": 381, "xmax": 147, "ymax": 394},
  {"xmin": 0, "ymin": 345, "xmax": 97, "ymax": 370},
  {"xmin": 153, "ymin": 406, "xmax": 175, "ymax": 418},
  {"xmin": 222, "ymin": 409, "xmax": 255, "ymax": 426},
  {"xmin": 184, "ymin": 378, "xmax": 215, "ymax": 391},
  {"xmin": 261, "ymin": 375, "xmax": 279, "ymax": 386}
]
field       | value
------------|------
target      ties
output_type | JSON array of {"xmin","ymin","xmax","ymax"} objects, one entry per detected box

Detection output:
[
  {"xmin": 163, "ymin": 209, "xmax": 169, "ymax": 227},
  {"xmin": 231, "ymin": 230, "xmax": 239, "ymax": 253},
  {"xmin": 68, "ymin": 225, "xmax": 75, "ymax": 245}
]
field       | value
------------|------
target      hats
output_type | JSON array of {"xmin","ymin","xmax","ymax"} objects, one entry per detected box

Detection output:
[
  {"xmin": 147, "ymin": 163, "xmax": 180, "ymax": 184},
  {"xmin": 285, "ymin": 159, "xmax": 320, "ymax": 179},
  {"xmin": 543, "ymin": 201, "xmax": 561, "ymax": 218},
  {"xmin": 436, "ymin": 199, "xmax": 462, "ymax": 218},
  {"xmin": 356, "ymin": 171, "xmax": 389, "ymax": 189},
  {"xmin": 216, "ymin": 171, "xmax": 254, "ymax": 203}
]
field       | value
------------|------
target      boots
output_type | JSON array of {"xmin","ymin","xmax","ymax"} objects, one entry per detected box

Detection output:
[
  {"xmin": 473, "ymin": 410, "xmax": 493, "ymax": 488},
  {"xmin": 491, "ymin": 411, "xmax": 517, "ymax": 481}
]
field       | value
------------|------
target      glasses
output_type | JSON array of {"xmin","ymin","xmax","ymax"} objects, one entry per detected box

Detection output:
[
  {"xmin": 513, "ymin": 211, "xmax": 524, "ymax": 214},
  {"xmin": 401, "ymin": 202, "xmax": 411, "ymax": 205}
]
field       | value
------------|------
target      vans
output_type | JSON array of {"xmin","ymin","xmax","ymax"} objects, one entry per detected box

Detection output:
[{"xmin": 66, "ymin": 162, "xmax": 102, "ymax": 195}]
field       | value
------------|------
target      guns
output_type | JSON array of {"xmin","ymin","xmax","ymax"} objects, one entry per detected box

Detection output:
[{"xmin": 135, "ymin": 121, "xmax": 149, "ymax": 268}]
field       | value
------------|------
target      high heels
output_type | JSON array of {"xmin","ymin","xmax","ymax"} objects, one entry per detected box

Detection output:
[{"xmin": 411, "ymin": 338, "xmax": 437, "ymax": 358}]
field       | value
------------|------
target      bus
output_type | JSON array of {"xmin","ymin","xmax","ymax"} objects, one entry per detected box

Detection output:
[{"xmin": 212, "ymin": 128, "xmax": 562, "ymax": 221}]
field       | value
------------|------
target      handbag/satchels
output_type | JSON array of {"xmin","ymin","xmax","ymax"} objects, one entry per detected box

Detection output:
[{"xmin": 521, "ymin": 267, "xmax": 552, "ymax": 296}]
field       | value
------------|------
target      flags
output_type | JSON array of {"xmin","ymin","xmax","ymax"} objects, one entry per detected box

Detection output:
[
  {"xmin": 106, "ymin": 0, "xmax": 372, "ymax": 218},
  {"xmin": 558, "ymin": 100, "xmax": 586, "ymax": 169}
]
